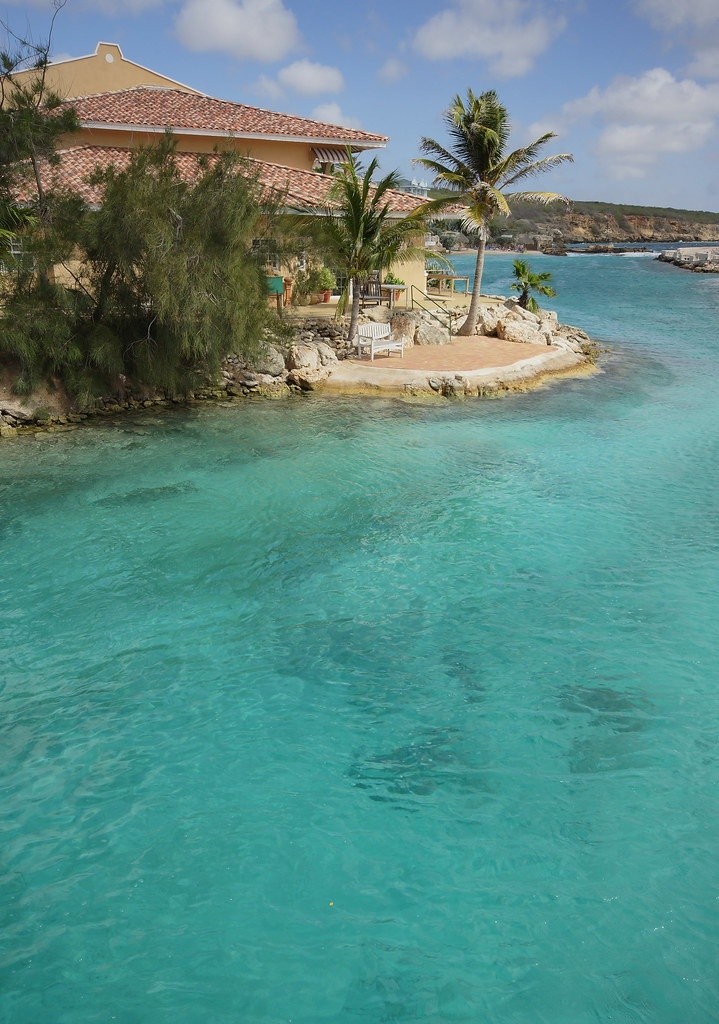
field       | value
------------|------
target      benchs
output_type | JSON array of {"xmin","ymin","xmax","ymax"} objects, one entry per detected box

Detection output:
[{"xmin": 357, "ymin": 321, "xmax": 404, "ymax": 362}]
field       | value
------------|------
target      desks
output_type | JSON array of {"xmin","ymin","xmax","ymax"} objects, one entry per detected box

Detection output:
[
  {"xmin": 380, "ymin": 284, "xmax": 408, "ymax": 310},
  {"xmin": 427, "ymin": 274, "xmax": 469, "ymax": 298}
]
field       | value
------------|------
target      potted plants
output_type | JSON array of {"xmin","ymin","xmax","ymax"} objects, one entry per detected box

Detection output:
[
  {"xmin": 379, "ymin": 271, "xmax": 405, "ymax": 301},
  {"xmin": 292, "ymin": 266, "xmax": 338, "ymax": 306}
]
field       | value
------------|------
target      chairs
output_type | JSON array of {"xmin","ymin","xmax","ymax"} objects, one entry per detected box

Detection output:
[{"xmin": 358, "ymin": 281, "xmax": 389, "ymax": 309}]
